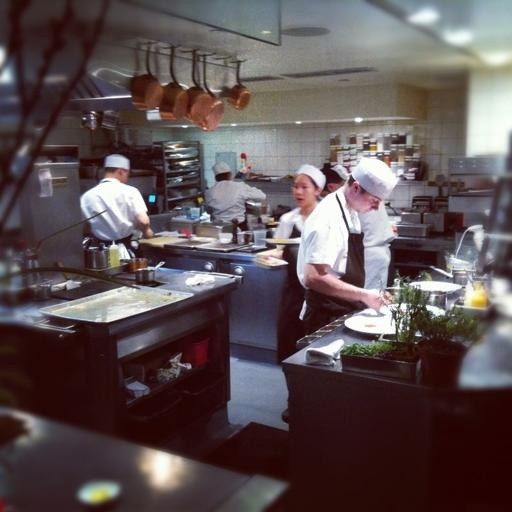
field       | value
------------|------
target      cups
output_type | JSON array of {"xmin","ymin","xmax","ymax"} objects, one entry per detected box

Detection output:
[
  {"xmin": 219, "ymin": 233, "xmax": 233, "ymax": 243},
  {"xmin": 254, "ymin": 230, "xmax": 267, "ymax": 248}
]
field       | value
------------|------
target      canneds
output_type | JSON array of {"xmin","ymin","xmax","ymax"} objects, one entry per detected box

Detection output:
[{"xmin": 390, "ymin": 143, "xmax": 422, "ymax": 163}]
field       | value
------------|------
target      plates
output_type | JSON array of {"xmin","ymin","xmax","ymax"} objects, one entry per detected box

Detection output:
[
  {"xmin": 344, "ymin": 303, "xmax": 445, "ymax": 333},
  {"xmin": 411, "ymin": 281, "xmax": 461, "ymax": 296},
  {"xmin": 263, "ymin": 238, "xmax": 301, "ymax": 245},
  {"xmin": 251, "ymin": 257, "xmax": 288, "ymax": 267},
  {"xmin": 76, "ymin": 477, "xmax": 121, "ymax": 506}
]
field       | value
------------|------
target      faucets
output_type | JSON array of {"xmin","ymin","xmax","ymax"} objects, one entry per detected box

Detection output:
[{"xmin": 468, "ymin": 223, "xmax": 487, "ymax": 237}]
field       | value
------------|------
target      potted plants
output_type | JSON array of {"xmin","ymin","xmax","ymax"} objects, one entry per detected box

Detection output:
[{"xmin": 340, "ymin": 270, "xmax": 487, "ymax": 387}]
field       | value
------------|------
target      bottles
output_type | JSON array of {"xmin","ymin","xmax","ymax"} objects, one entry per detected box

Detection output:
[
  {"xmin": 470, "ymin": 280, "xmax": 487, "ymax": 308},
  {"xmin": 16, "ymin": 240, "xmax": 40, "ymax": 271},
  {"xmin": 240, "ymin": 154, "xmax": 246, "ymax": 170},
  {"xmin": 108, "ymin": 240, "xmax": 120, "ymax": 266},
  {"xmin": 328, "ymin": 132, "xmax": 421, "ymax": 180}
]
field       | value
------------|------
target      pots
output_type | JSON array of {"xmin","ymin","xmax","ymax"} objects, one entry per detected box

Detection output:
[
  {"xmin": 130, "ymin": 42, "xmax": 251, "ymax": 131},
  {"xmin": 245, "ymin": 199, "xmax": 267, "ymax": 216},
  {"xmin": 236, "ymin": 226, "xmax": 253, "ymax": 244},
  {"xmin": 120, "ymin": 257, "xmax": 148, "ymax": 272},
  {"xmin": 85, "ymin": 242, "xmax": 108, "ymax": 268},
  {"xmin": 136, "ymin": 261, "xmax": 165, "ymax": 282}
]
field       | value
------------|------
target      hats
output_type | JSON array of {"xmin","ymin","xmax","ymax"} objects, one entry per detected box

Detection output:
[
  {"xmin": 352, "ymin": 158, "xmax": 398, "ymax": 201},
  {"xmin": 329, "ymin": 165, "xmax": 350, "ymax": 181},
  {"xmin": 211, "ymin": 160, "xmax": 231, "ymax": 176},
  {"xmin": 104, "ymin": 154, "xmax": 131, "ymax": 170},
  {"xmin": 294, "ymin": 164, "xmax": 327, "ymax": 192}
]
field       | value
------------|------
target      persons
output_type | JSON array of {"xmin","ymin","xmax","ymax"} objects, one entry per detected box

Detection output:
[
  {"xmin": 203, "ymin": 160, "xmax": 267, "ymax": 242},
  {"xmin": 77, "ymin": 151, "xmax": 157, "ymax": 261},
  {"xmin": 255, "ymin": 156, "xmax": 402, "ymax": 424}
]
